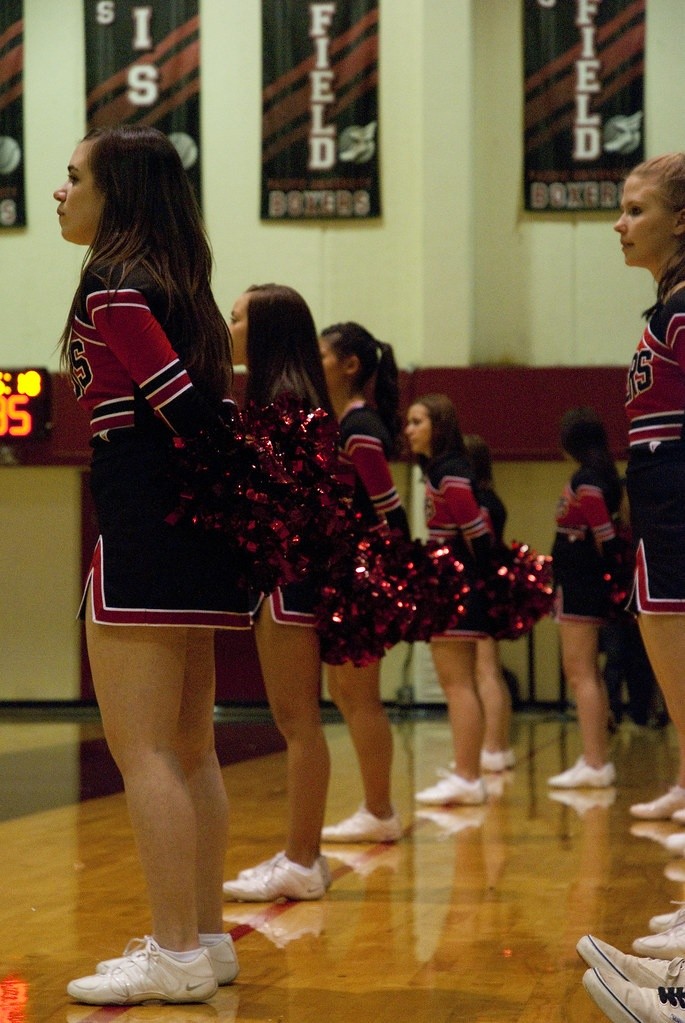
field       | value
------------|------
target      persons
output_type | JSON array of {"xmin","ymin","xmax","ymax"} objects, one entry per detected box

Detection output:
[
  {"xmin": 224, "ymin": 282, "xmax": 332, "ymax": 900},
  {"xmin": 407, "ymin": 397, "xmax": 515, "ymax": 802},
  {"xmin": 320, "ymin": 322, "xmax": 403, "ymax": 842},
  {"xmin": 113, "ymin": 788, "xmax": 620, "ymax": 1022},
  {"xmin": 548, "ymin": 154, "xmax": 685, "ymax": 1023},
  {"xmin": 53, "ymin": 130, "xmax": 238, "ymax": 1001}
]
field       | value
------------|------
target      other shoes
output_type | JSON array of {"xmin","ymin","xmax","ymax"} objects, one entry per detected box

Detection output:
[
  {"xmin": 448, "ymin": 749, "xmax": 518, "ymax": 772},
  {"xmin": 630, "ymin": 785, "xmax": 685, "ymax": 818},
  {"xmin": 484, "ymin": 770, "xmax": 517, "ymax": 797},
  {"xmin": 547, "ymin": 755, "xmax": 617, "ymax": 788},
  {"xmin": 546, "ymin": 788, "xmax": 616, "ymax": 815},
  {"xmin": 630, "ymin": 821, "xmax": 685, "ymax": 850}
]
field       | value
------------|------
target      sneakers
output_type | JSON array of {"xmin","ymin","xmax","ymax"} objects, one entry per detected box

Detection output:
[
  {"xmin": 632, "ymin": 924, "xmax": 685, "ymax": 960},
  {"xmin": 96, "ymin": 929, "xmax": 240, "ymax": 983},
  {"xmin": 582, "ymin": 966, "xmax": 685, "ymax": 1023},
  {"xmin": 414, "ymin": 807, "xmax": 488, "ymax": 840},
  {"xmin": 66, "ymin": 935, "xmax": 219, "ymax": 1004},
  {"xmin": 576, "ymin": 935, "xmax": 685, "ymax": 989},
  {"xmin": 239, "ymin": 851, "xmax": 329, "ymax": 888},
  {"xmin": 320, "ymin": 808, "xmax": 402, "ymax": 842},
  {"xmin": 649, "ymin": 899, "xmax": 685, "ymax": 932},
  {"xmin": 223, "ymin": 854, "xmax": 326, "ymax": 900},
  {"xmin": 415, "ymin": 773, "xmax": 484, "ymax": 804},
  {"xmin": 221, "ymin": 901, "xmax": 325, "ymax": 948},
  {"xmin": 318, "ymin": 844, "xmax": 407, "ymax": 874}
]
